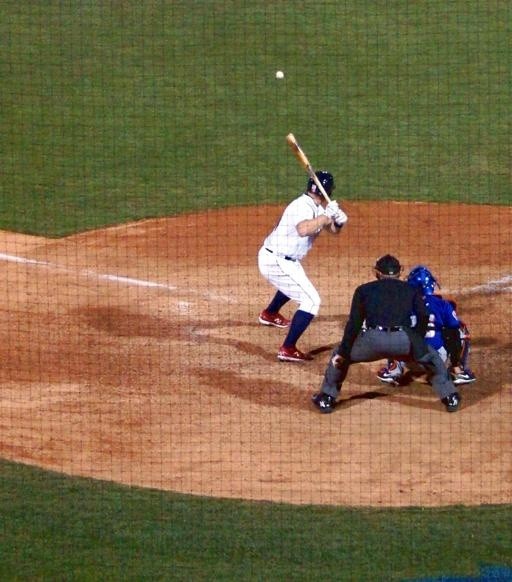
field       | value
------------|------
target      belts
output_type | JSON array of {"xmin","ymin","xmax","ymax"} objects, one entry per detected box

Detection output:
[
  {"xmin": 264, "ymin": 247, "xmax": 296, "ymax": 262},
  {"xmin": 370, "ymin": 324, "xmax": 405, "ymax": 332}
]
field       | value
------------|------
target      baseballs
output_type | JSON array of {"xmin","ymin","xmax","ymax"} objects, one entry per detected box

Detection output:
[{"xmin": 275, "ymin": 71, "xmax": 285, "ymax": 80}]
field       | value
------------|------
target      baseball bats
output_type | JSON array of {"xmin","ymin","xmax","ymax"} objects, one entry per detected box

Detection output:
[{"xmin": 285, "ymin": 133, "xmax": 333, "ymax": 204}]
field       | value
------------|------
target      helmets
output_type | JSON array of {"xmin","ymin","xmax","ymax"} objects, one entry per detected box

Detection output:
[
  {"xmin": 406, "ymin": 266, "xmax": 437, "ymax": 295},
  {"xmin": 307, "ymin": 171, "xmax": 334, "ymax": 196},
  {"xmin": 376, "ymin": 253, "xmax": 401, "ymax": 275}
]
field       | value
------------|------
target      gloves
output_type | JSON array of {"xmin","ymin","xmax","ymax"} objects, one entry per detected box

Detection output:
[{"xmin": 327, "ymin": 200, "xmax": 348, "ymax": 225}]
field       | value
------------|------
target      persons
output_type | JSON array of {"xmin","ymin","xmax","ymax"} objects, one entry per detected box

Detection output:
[
  {"xmin": 258, "ymin": 171, "xmax": 348, "ymax": 361},
  {"xmin": 311, "ymin": 254, "xmax": 461, "ymax": 413},
  {"xmin": 377, "ymin": 266, "xmax": 476, "ymax": 383}
]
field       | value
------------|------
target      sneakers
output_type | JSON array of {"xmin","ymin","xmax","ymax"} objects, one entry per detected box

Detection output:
[
  {"xmin": 440, "ymin": 392, "xmax": 461, "ymax": 413},
  {"xmin": 377, "ymin": 369, "xmax": 403, "ymax": 386},
  {"xmin": 259, "ymin": 310, "xmax": 292, "ymax": 328},
  {"xmin": 277, "ymin": 345, "xmax": 313, "ymax": 362},
  {"xmin": 312, "ymin": 394, "xmax": 335, "ymax": 413},
  {"xmin": 448, "ymin": 370, "xmax": 477, "ymax": 384}
]
